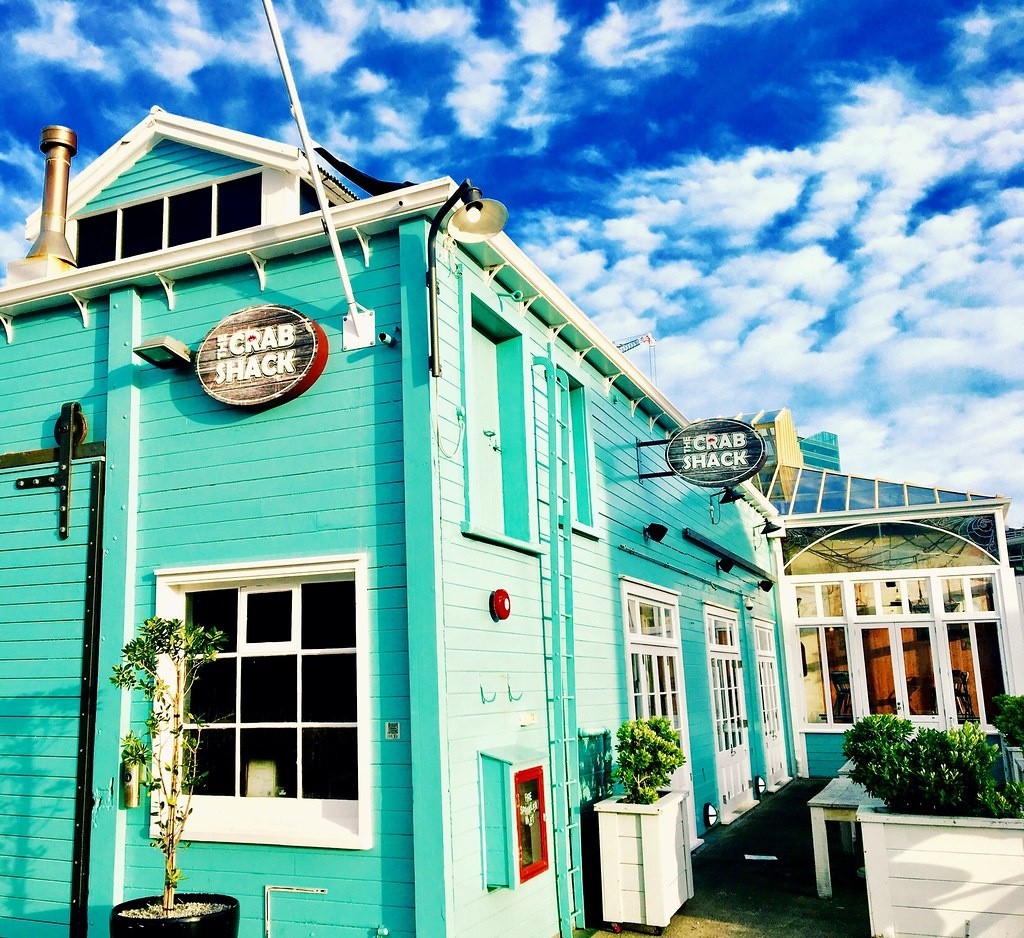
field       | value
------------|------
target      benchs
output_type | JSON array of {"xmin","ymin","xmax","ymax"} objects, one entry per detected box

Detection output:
[
  {"xmin": 839, "ymin": 754, "xmax": 880, "ymax": 842},
  {"xmin": 806, "ymin": 778, "xmax": 890, "ymax": 899}
]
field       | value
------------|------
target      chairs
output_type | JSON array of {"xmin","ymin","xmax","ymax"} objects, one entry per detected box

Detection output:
[
  {"xmin": 930, "ymin": 669, "xmax": 977, "ymax": 722},
  {"xmin": 818, "ymin": 670, "xmax": 854, "ymax": 723}
]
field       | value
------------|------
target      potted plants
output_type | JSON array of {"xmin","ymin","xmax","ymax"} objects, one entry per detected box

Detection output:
[
  {"xmin": 592, "ymin": 716, "xmax": 694, "ymax": 928},
  {"xmin": 108, "ymin": 611, "xmax": 240, "ymax": 938}
]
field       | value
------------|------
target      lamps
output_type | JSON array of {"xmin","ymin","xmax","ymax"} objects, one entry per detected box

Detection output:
[
  {"xmin": 753, "ymin": 518, "xmax": 782, "ymax": 550},
  {"xmin": 642, "ymin": 522, "xmax": 667, "ymax": 542},
  {"xmin": 912, "ymin": 580, "xmax": 930, "ymax": 613},
  {"xmin": 758, "ymin": 580, "xmax": 773, "ymax": 593},
  {"xmin": 702, "ymin": 802, "xmax": 718, "ymax": 829},
  {"xmin": 944, "ymin": 580, "xmax": 960, "ymax": 613},
  {"xmin": 715, "ymin": 558, "xmax": 735, "ymax": 573},
  {"xmin": 132, "ymin": 335, "xmax": 192, "ymax": 371},
  {"xmin": 426, "ymin": 177, "xmax": 510, "ymax": 378},
  {"xmin": 890, "ymin": 587, "xmax": 903, "ymax": 606},
  {"xmin": 755, "ymin": 775, "xmax": 767, "ymax": 795},
  {"xmin": 710, "ymin": 487, "xmax": 746, "ymax": 524},
  {"xmin": 855, "ymin": 585, "xmax": 867, "ymax": 607}
]
